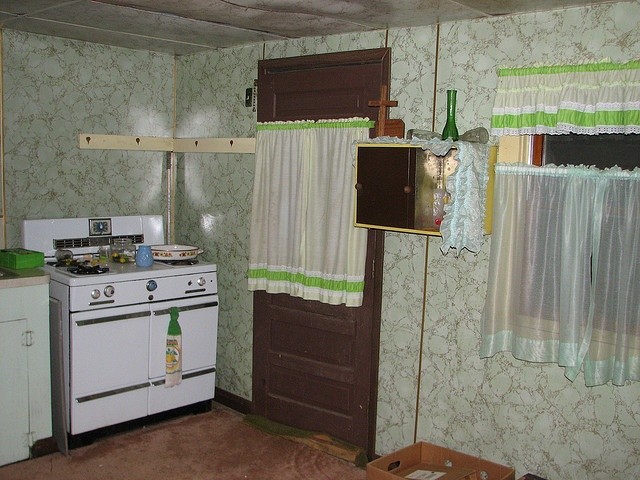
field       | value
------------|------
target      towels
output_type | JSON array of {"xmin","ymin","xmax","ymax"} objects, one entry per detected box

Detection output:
[{"xmin": 164, "ymin": 306, "xmax": 183, "ymax": 387}]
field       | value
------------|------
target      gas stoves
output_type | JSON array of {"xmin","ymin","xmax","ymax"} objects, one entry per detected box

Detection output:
[{"xmin": 42, "ymin": 249, "xmax": 215, "ymax": 280}]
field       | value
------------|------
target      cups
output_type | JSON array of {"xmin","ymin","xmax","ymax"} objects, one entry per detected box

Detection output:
[{"xmin": 135, "ymin": 245, "xmax": 154, "ymax": 267}]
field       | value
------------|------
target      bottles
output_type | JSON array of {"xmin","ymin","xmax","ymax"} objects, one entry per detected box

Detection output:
[
  {"xmin": 111, "ymin": 238, "xmax": 133, "ymax": 263},
  {"xmin": 406, "ymin": 128, "xmax": 443, "ymax": 141},
  {"xmin": 459, "ymin": 127, "xmax": 490, "ymax": 145}
]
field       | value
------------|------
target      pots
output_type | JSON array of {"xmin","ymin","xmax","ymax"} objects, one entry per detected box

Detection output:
[{"xmin": 148, "ymin": 243, "xmax": 204, "ymax": 261}]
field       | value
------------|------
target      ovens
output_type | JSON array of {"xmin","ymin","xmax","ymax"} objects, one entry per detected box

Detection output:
[{"xmin": 70, "ymin": 296, "xmax": 220, "ymax": 436}]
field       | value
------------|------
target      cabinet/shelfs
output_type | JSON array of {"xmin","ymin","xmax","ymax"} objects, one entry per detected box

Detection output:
[
  {"xmin": 352, "ymin": 141, "xmax": 497, "ymax": 240},
  {"xmin": 1, "ymin": 282, "xmax": 53, "ymax": 467}
]
